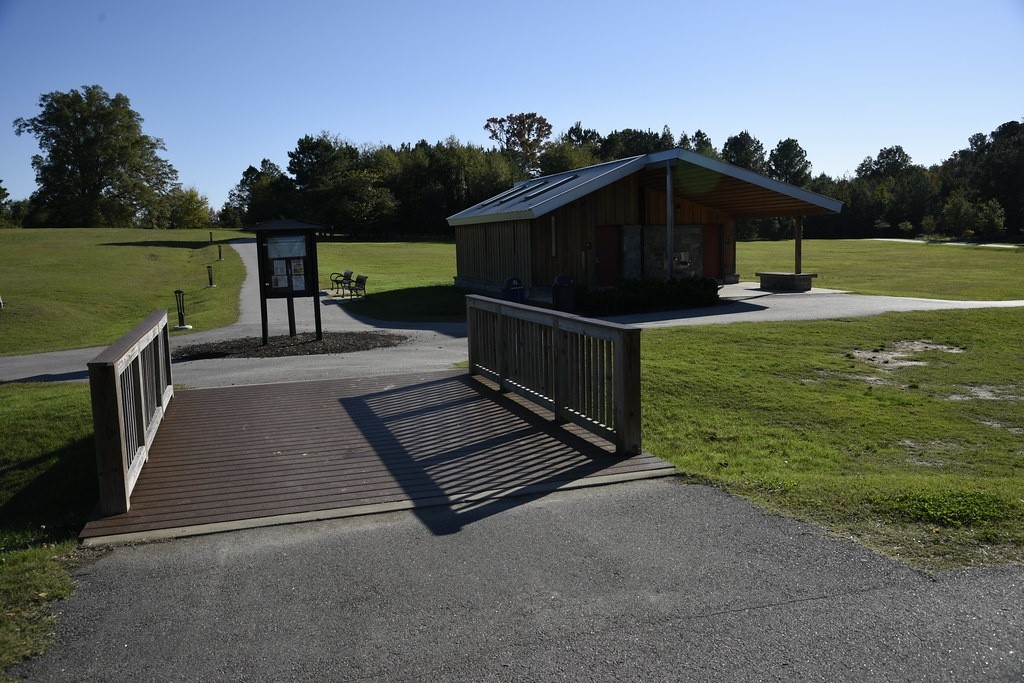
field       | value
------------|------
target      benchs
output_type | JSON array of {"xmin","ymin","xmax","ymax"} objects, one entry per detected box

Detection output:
[
  {"xmin": 341, "ymin": 275, "xmax": 368, "ymax": 301},
  {"xmin": 330, "ymin": 269, "xmax": 353, "ymax": 294}
]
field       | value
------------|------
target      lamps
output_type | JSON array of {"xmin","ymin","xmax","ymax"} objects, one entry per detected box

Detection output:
[
  {"xmin": 209, "ymin": 232, "xmax": 212, "ymax": 237},
  {"xmin": 217, "ymin": 244, "xmax": 220, "ymax": 253},
  {"xmin": 206, "ymin": 265, "xmax": 212, "ymax": 278},
  {"xmin": 173, "ymin": 289, "xmax": 184, "ymax": 313}
]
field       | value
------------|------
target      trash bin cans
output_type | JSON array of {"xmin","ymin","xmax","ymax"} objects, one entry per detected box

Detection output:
[
  {"xmin": 501, "ymin": 277, "xmax": 524, "ymax": 304},
  {"xmin": 551, "ymin": 274, "xmax": 575, "ymax": 313}
]
